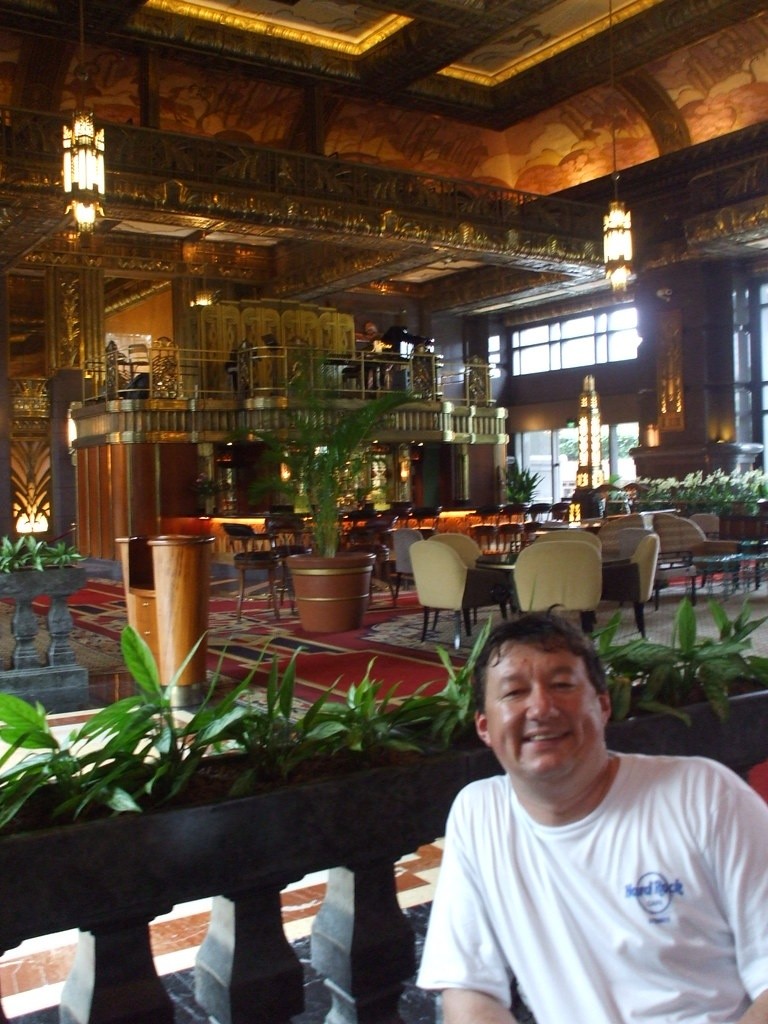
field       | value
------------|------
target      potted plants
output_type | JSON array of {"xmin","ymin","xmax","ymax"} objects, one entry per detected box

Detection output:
[{"xmin": 238, "ymin": 344, "xmax": 432, "ymax": 636}]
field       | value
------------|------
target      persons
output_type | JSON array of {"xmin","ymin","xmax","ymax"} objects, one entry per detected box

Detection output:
[{"xmin": 415, "ymin": 613, "xmax": 768, "ymax": 1024}]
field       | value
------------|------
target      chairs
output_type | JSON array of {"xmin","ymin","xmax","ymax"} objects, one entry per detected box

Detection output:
[{"xmin": 223, "ymin": 501, "xmax": 739, "ymax": 643}]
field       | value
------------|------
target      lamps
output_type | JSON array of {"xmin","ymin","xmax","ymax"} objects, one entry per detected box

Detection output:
[
  {"xmin": 62, "ymin": 0, "xmax": 108, "ymax": 226},
  {"xmin": 601, "ymin": 0, "xmax": 635, "ymax": 293}
]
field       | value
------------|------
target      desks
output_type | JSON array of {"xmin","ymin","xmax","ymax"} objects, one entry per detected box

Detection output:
[
  {"xmin": 532, "ymin": 521, "xmax": 606, "ymax": 534},
  {"xmin": 474, "ymin": 548, "xmax": 631, "ymax": 633}
]
